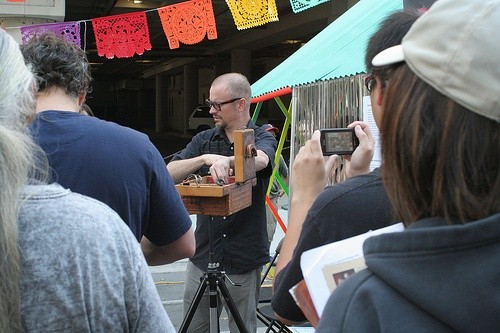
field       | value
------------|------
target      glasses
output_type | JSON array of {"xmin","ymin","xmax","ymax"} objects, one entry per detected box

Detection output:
[
  {"xmin": 364, "ymin": 67, "xmax": 388, "ymax": 92},
  {"xmin": 205, "ymin": 97, "xmax": 242, "ymax": 112}
]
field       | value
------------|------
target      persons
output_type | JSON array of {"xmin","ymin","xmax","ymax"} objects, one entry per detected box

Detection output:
[
  {"xmin": 21, "ymin": 31, "xmax": 195, "ymax": 266},
  {"xmin": 262, "ymin": 124, "xmax": 288, "ymax": 267},
  {"xmin": 0, "ymin": 27, "xmax": 175, "ymax": 333},
  {"xmin": 272, "ymin": 9, "xmax": 419, "ymax": 325},
  {"xmin": 316, "ymin": 0, "xmax": 500, "ymax": 333},
  {"xmin": 165, "ymin": 72, "xmax": 277, "ymax": 333}
]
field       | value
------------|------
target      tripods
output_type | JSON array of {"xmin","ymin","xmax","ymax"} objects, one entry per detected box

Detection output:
[{"xmin": 178, "ymin": 215, "xmax": 249, "ymax": 333}]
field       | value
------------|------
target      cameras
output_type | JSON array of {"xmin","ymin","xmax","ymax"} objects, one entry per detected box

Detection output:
[{"xmin": 320, "ymin": 127, "xmax": 359, "ymax": 155}]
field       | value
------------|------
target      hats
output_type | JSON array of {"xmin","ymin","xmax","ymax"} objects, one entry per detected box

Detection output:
[
  {"xmin": 261, "ymin": 123, "xmax": 280, "ymax": 133},
  {"xmin": 371, "ymin": 0, "xmax": 500, "ymax": 126}
]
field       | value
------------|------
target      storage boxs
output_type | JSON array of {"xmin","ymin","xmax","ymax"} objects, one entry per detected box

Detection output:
[{"xmin": 173, "ymin": 128, "xmax": 258, "ymax": 217}]
